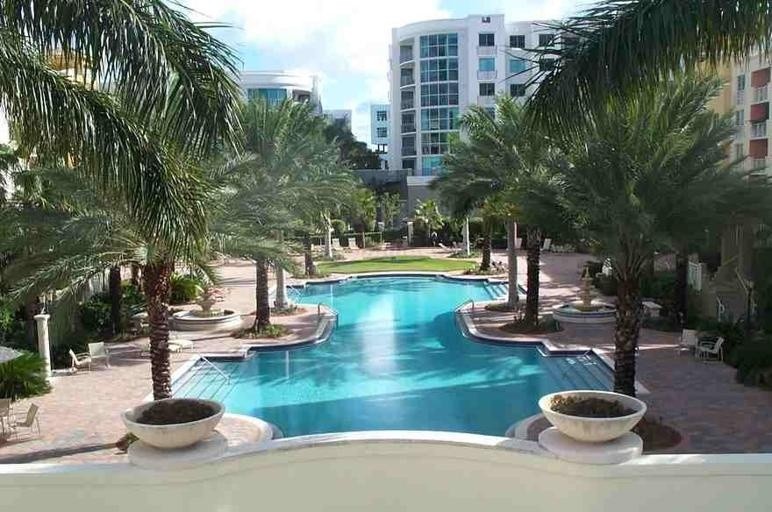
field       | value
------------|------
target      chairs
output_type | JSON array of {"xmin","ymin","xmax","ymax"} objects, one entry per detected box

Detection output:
[
  {"xmin": 310, "ymin": 236, "xmax": 360, "ymax": 255},
  {"xmin": 438, "ymin": 236, "xmax": 552, "ymax": 257},
  {"xmin": 673, "ymin": 325, "xmax": 725, "ymax": 364},
  {"xmin": 0, "ymin": 333, "xmax": 195, "ymax": 448}
]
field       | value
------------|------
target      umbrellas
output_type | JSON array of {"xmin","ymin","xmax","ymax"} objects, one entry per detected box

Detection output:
[{"xmin": 0, "ymin": 346, "xmax": 23, "ymax": 364}]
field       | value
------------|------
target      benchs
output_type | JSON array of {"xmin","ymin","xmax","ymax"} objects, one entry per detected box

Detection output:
[{"xmin": 640, "ymin": 299, "xmax": 664, "ymax": 317}]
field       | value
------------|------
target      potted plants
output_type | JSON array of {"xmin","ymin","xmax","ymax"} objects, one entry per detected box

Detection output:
[
  {"xmin": 537, "ymin": 387, "xmax": 647, "ymax": 443},
  {"xmin": 121, "ymin": 395, "xmax": 228, "ymax": 451}
]
